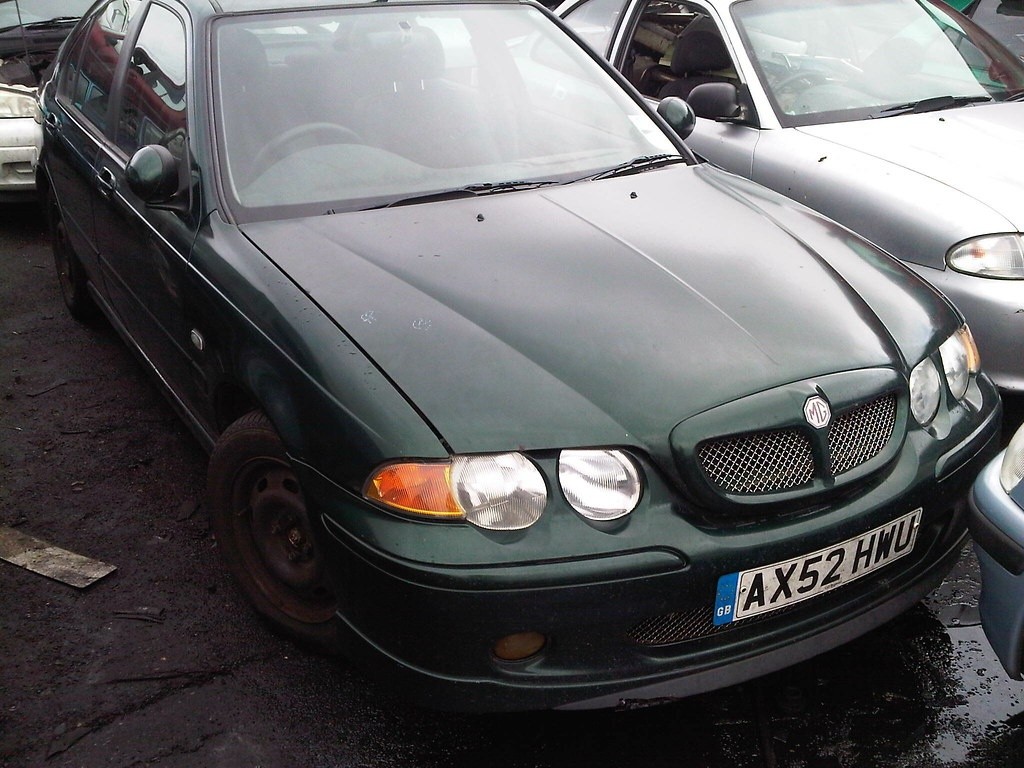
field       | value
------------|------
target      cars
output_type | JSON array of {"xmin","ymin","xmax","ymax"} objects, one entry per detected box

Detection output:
[
  {"xmin": 1, "ymin": 0, "xmax": 97, "ymax": 189},
  {"xmin": 472, "ymin": 0, "xmax": 1024, "ymax": 398},
  {"xmin": 31, "ymin": 0, "xmax": 1004, "ymax": 715}
]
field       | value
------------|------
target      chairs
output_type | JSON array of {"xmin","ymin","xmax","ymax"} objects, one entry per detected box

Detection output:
[
  {"xmin": 367, "ymin": 24, "xmax": 492, "ymax": 170},
  {"xmin": 217, "ymin": 28, "xmax": 286, "ymax": 180},
  {"xmin": 658, "ymin": 29, "xmax": 740, "ymax": 104}
]
